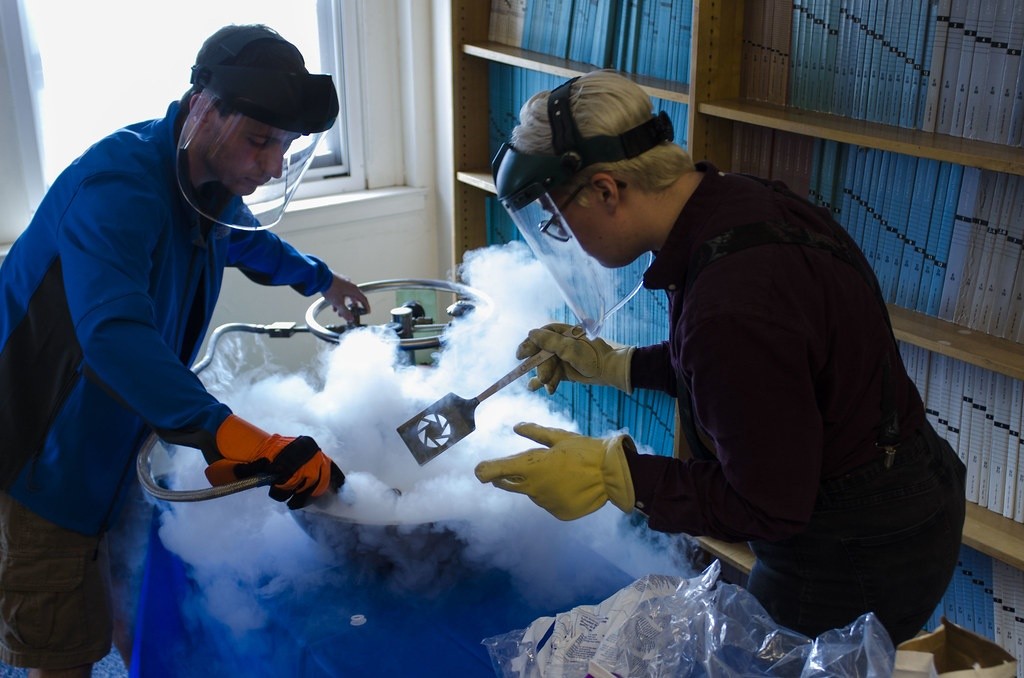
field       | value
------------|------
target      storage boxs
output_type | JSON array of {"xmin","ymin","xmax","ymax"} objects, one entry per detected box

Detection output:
[{"xmin": 893, "ymin": 615, "xmax": 1017, "ymax": 678}]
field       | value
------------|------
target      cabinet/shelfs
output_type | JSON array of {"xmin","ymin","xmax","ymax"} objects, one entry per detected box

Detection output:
[{"xmin": 450, "ymin": 0, "xmax": 1024, "ymax": 638}]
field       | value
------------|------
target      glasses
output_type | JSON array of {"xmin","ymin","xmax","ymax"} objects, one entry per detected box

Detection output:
[{"xmin": 537, "ymin": 179, "xmax": 628, "ymax": 243}]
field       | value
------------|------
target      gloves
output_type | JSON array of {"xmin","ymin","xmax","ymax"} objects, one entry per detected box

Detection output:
[
  {"xmin": 474, "ymin": 422, "xmax": 639, "ymax": 522},
  {"xmin": 515, "ymin": 323, "xmax": 637, "ymax": 396},
  {"xmin": 204, "ymin": 413, "xmax": 345, "ymax": 510}
]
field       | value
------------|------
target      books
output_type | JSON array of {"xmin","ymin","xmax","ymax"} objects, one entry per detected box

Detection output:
[{"xmin": 471, "ymin": 0, "xmax": 1023, "ymax": 677}]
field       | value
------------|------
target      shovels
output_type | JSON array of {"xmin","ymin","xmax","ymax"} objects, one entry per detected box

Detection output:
[{"xmin": 396, "ymin": 323, "xmax": 589, "ymax": 468}]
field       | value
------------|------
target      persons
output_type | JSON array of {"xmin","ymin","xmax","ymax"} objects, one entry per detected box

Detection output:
[
  {"xmin": 469, "ymin": 70, "xmax": 969, "ymax": 677},
  {"xmin": 0, "ymin": 24, "xmax": 371, "ymax": 678}
]
